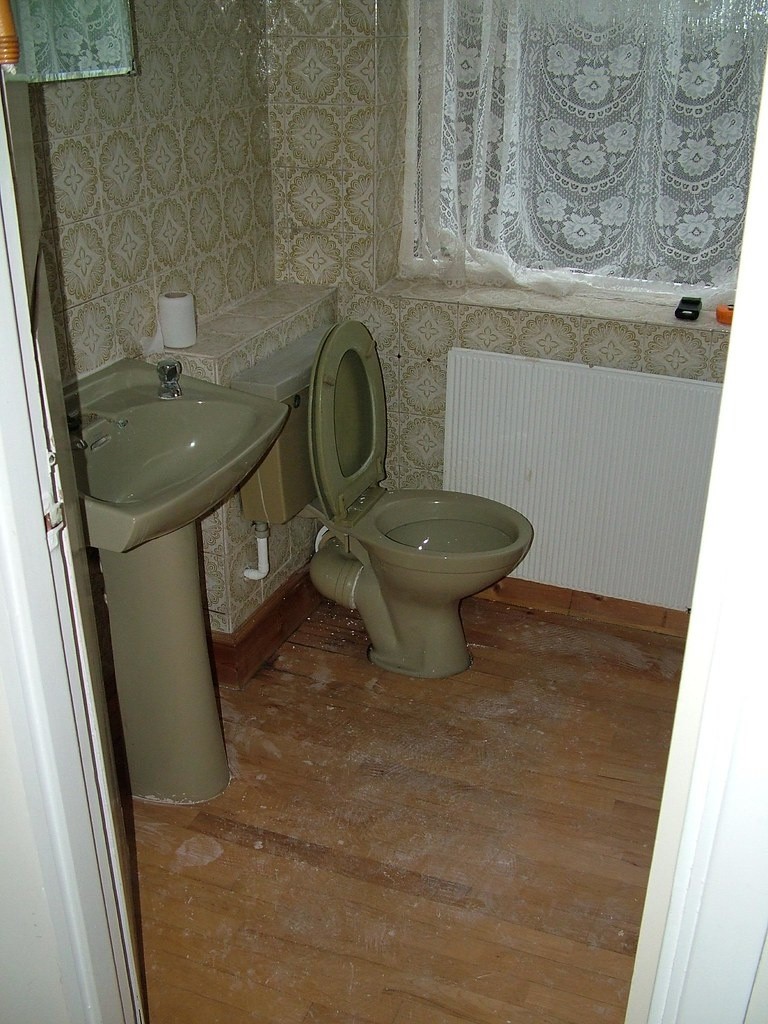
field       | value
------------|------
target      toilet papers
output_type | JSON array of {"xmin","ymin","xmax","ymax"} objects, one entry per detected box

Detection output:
[{"xmin": 139, "ymin": 289, "xmax": 197, "ymax": 358}]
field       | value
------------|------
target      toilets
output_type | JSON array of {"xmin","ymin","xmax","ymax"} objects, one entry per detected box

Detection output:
[{"xmin": 229, "ymin": 317, "xmax": 535, "ymax": 679}]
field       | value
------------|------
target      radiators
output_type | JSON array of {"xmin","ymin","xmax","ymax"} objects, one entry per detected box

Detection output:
[{"xmin": 441, "ymin": 344, "xmax": 726, "ymax": 617}]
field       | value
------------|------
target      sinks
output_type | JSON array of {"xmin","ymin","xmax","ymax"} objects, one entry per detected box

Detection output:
[{"xmin": 60, "ymin": 356, "xmax": 292, "ymax": 555}]
